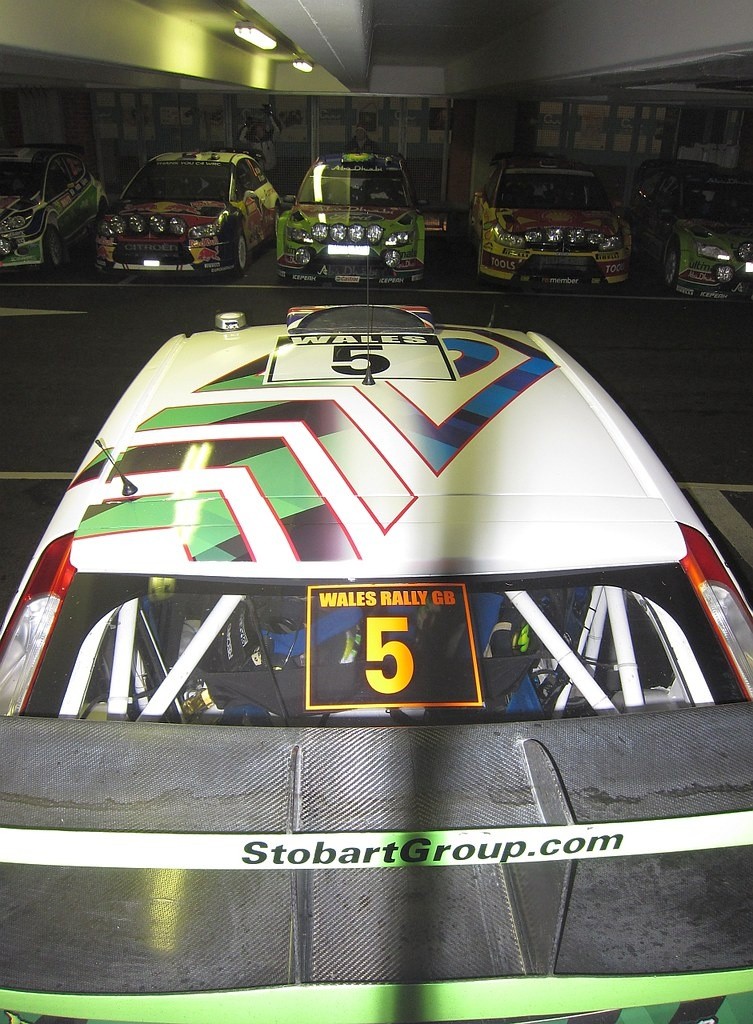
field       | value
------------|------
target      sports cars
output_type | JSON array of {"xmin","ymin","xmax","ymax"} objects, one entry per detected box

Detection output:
[{"xmin": 0, "ymin": 301, "xmax": 753, "ymax": 1017}]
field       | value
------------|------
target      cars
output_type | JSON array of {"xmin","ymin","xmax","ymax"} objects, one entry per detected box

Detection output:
[
  {"xmin": 91, "ymin": 150, "xmax": 283, "ymax": 284},
  {"xmin": 473, "ymin": 153, "xmax": 632, "ymax": 295},
  {"xmin": 631, "ymin": 157, "xmax": 753, "ymax": 302},
  {"xmin": 0, "ymin": 149, "xmax": 108, "ymax": 276},
  {"xmin": 276, "ymin": 152, "xmax": 427, "ymax": 291}
]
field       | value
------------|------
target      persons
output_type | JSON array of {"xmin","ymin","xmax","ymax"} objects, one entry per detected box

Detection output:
[
  {"xmin": 347, "ymin": 121, "xmax": 380, "ymax": 153},
  {"xmin": 237, "ymin": 102, "xmax": 284, "ymax": 177}
]
